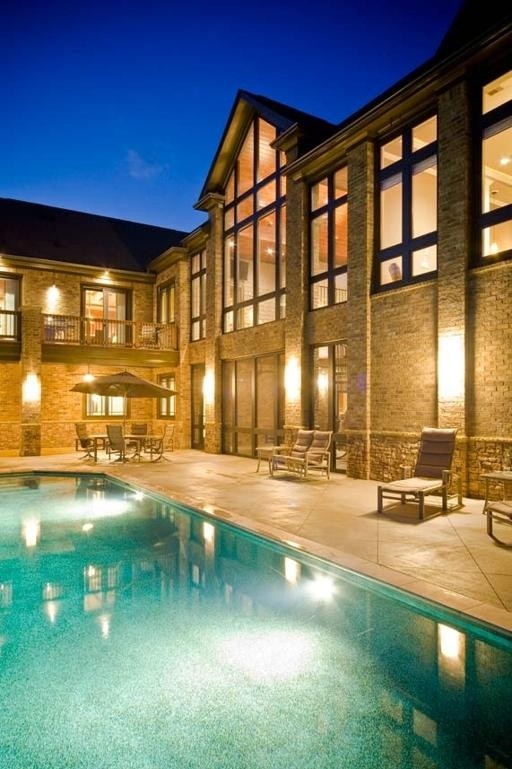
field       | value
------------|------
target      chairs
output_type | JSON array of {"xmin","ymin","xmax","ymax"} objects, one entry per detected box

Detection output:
[
  {"xmin": 486, "ymin": 501, "xmax": 511, "ymax": 534},
  {"xmin": 287, "ymin": 431, "xmax": 332, "ymax": 480},
  {"xmin": 75, "ymin": 423, "xmax": 175, "ymax": 463},
  {"xmin": 378, "ymin": 428, "xmax": 462, "ymax": 519},
  {"xmin": 271, "ymin": 429, "xmax": 312, "ymax": 479}
]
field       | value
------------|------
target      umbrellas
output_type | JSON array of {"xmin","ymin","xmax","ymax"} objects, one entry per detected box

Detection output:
[{"xmin": 67, "ymin": 371, "xmax": 181, "ymax": 436}]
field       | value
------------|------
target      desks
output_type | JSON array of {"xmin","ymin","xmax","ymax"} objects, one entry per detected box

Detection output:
[
  {"xmin": 255, "ymin": 446, "xmax": 288, "ymax": 474},
  {"xmin": 479, "ymin": 471, "xmax": 512, "ymax": 512}
]
